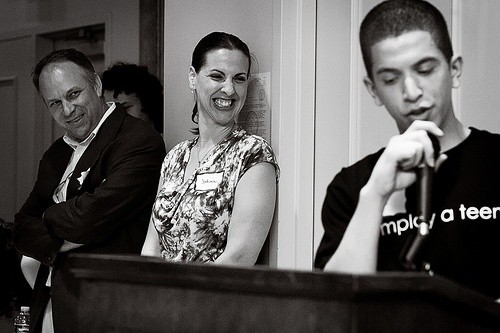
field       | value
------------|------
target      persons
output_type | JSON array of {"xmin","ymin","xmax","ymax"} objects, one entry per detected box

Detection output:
[
  {"xmin": 314, "ymin": 0, "xmax": 500, "ymax": 303},
  {"xmin": 2, "ymin": 48, "xmax": 166, "ymax": 333},
  {"xmin": 140, "ymin": 32, "xmax": 280, "ymax": 268},
  {"xmin": 101, "ymin": 60, "xmax": 164, "ymax": 138}
]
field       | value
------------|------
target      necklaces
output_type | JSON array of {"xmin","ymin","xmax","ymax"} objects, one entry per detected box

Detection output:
[{"xmin": 197, "ymin": 128, "xmax": 233, "ymax": 169}]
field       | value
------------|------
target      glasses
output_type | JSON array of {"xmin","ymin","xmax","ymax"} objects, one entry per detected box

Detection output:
[{"xmin": 55, "ymin": 171, "xmax": 75, "ymax": 203}]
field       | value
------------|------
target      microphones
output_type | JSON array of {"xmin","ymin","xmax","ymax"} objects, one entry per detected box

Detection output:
[{"xmin": 416, "ymin": 130, "xmax": 442, "ymax": 236}]
